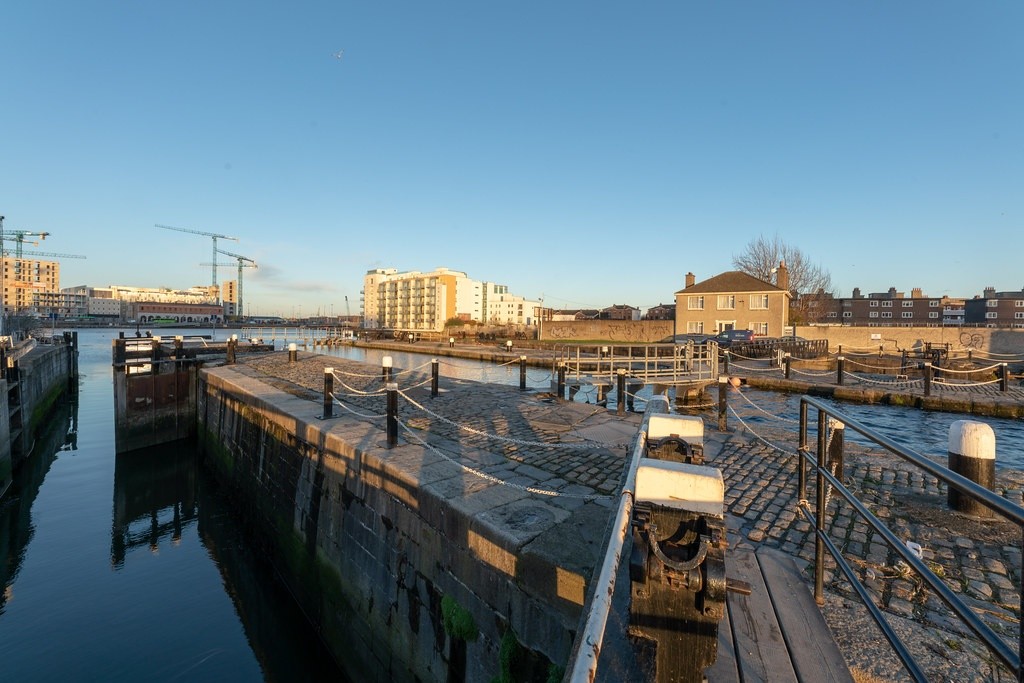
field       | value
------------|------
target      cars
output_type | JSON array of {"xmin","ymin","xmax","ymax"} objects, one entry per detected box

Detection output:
[{"xmin": 716, "ymin": 331, "xmax": 754, "ymax": 343}]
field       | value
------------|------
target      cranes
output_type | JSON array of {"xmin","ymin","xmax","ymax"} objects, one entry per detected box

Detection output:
[
  {"xmin": 0, "ymin": 215, "xmax": 87, "ymax": 259},
  {"xmin": 155, "ymin": 223, "xmax": 239, "ymax": 286},
  {"xmin": 200, "ymin": 249, "xmax": 258, "ymax": 320}
]
